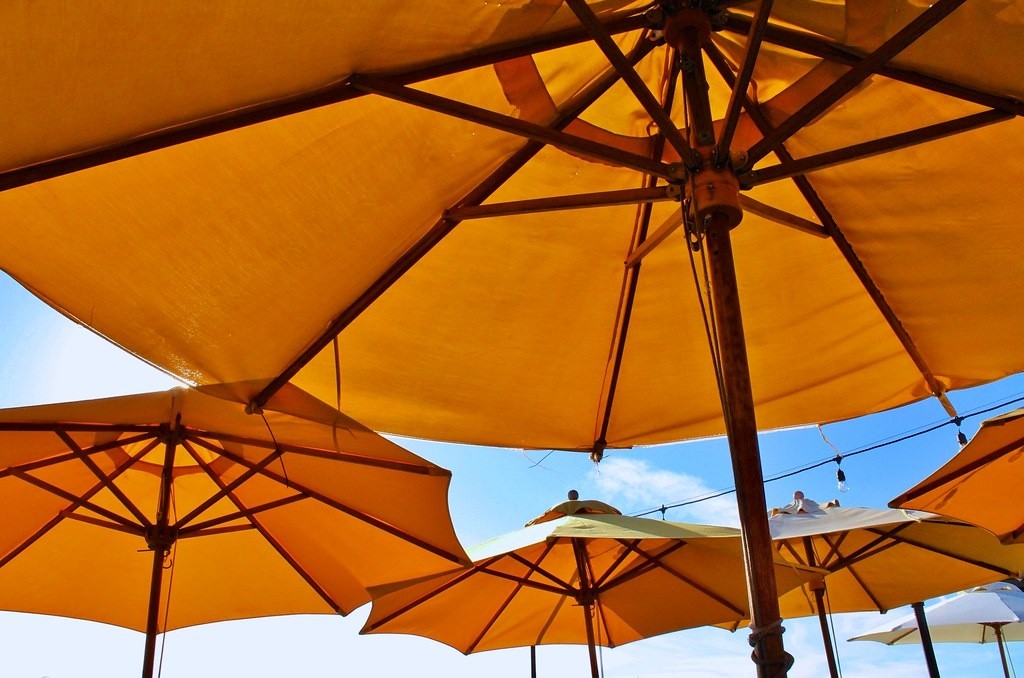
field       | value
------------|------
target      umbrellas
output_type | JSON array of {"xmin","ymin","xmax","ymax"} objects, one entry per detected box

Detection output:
[
  {"xmin": 0, "ymin": 0, "xmax": 1024, "ymax": 678},
  {"xmin": 848, "ymin": 580, "xmax": 1024, "ymax": 678},
  {"xmin": 708, "ymin": 490, "xmax": 1024, "ymax": 678},
  {"xmin": 359, "ymin": 487, "xmax": 831, "ymax": 678},
  {"xmin": 888, "ymin": 405, "xmax": 1024, "ymax": 545},
  {"xmin": 0, "ymin": 378, "xmax": 476, "ymax": 678}
]
